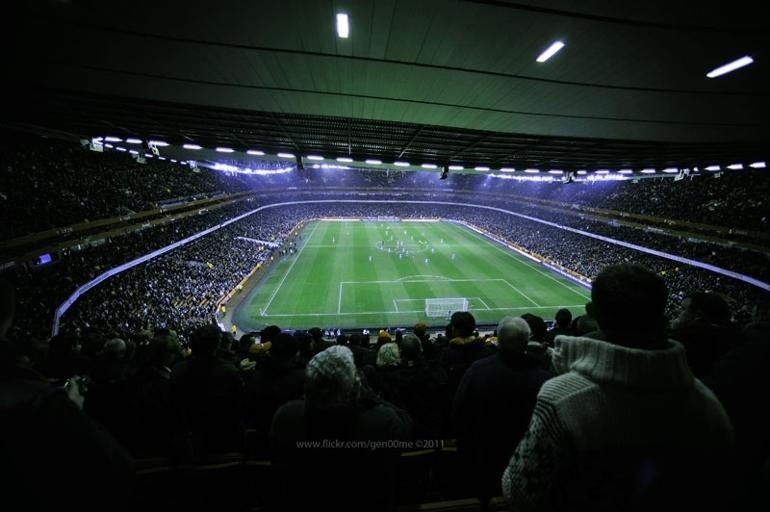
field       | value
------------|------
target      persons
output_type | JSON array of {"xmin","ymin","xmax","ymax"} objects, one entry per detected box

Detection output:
[
  {"xmin": 273, "ymin": 310, "xmax": 770, "ymax": 512},
  {"xmin": 272, "ymin": 161, "xmax": 769, "ymax": 263},
  {"xmin": 2, "ymin": 138, "xmax": 272, "ymax": 509},
  {"xmin": 552, "ymin": 262, "xmax": 769, "ymax": 311}
]
